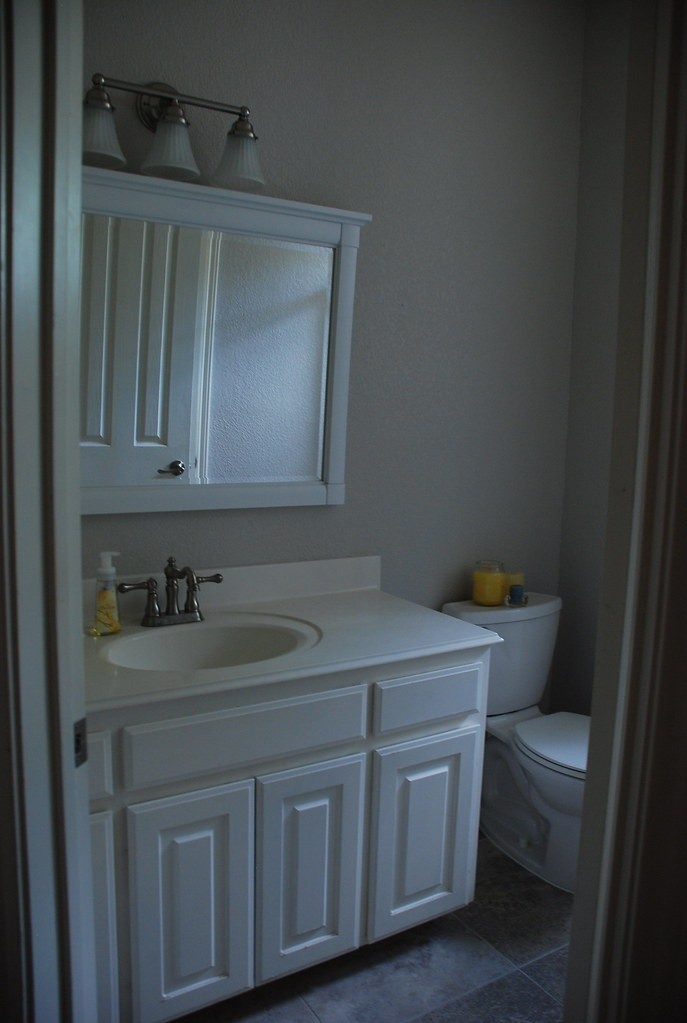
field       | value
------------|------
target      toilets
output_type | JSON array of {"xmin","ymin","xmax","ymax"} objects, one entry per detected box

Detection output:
[{"xmin": 441, "ymin": 590, "xmax": 593, "ymax": 896}]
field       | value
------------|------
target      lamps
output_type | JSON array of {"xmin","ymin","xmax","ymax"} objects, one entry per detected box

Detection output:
[{"xmin": 81, "ymin": 70, "xmax": 265, "ymax": 191}]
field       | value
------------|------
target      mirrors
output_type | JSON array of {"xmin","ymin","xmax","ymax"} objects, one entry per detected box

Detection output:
[{"xmin": 78, "ymin": 165, "xmax": 373, "ymax": 512}]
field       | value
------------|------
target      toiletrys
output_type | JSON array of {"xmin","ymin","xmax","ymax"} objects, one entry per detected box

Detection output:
[{"xmin": 95, "ymin": 551, "xmax": 122, "ymax": 635}]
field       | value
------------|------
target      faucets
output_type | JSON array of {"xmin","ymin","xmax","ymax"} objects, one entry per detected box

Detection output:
[{"xmin": 163, "ymin": 557, "xmax": 200, "ymax": 616}]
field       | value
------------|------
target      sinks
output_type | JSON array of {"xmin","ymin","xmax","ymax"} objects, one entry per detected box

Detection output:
[{"xmin": 104, "ymin": 608, "xmax": 327, "ymax": 670}]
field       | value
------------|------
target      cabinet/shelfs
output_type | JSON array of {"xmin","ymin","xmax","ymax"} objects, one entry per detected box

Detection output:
[{"xmin": 77, "ymin": 645, "xmax": 492, "ymax": 1023}]
